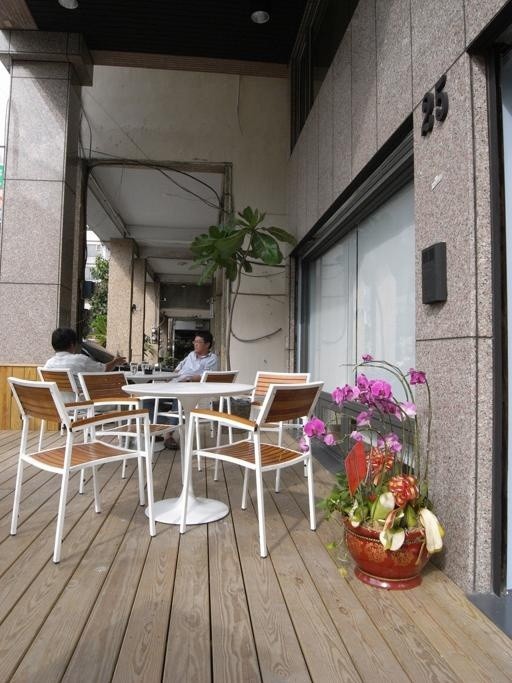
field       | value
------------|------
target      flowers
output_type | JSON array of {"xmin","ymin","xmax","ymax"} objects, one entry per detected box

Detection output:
[{"xmin": 276, "ymin": 353, "xmax": 445, "ymax": 576}]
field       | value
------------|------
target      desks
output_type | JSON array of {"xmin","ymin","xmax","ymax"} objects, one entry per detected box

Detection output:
[
  {"xmin": 108, "ymin": 370, "xmax": 180, "ymax": 451},
  {"xmin": 123, "ymin": 380, "xmax": 253, "ymax": 525}
]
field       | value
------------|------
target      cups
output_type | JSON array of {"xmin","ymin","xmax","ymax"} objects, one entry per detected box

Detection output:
[
  {"xmin": 130, "ymin": 362, "xmax": 139, "ymax": 376},
  {"xmin": 154, "ymin": 362, "xmax": 162, "ymax": 373}
]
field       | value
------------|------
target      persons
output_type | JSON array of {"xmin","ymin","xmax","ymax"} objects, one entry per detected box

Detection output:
[
  {"xmin": 45, "ymin": 327, "xmax": 133, "ymax": 449},
  {"xmin": 144, "ymin": 329, "xmax": 221, "ymax": 449}
]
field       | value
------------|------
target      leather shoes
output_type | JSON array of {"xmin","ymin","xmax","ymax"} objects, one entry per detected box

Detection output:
[{"xmin": 163, "ymin": 436, "xmax": 179, "ymax": 450}]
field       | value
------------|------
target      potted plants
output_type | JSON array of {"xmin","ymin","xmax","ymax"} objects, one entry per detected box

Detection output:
[{"xmin": 185, "ymin": 204, "xmax": 298, "ymax": 436}]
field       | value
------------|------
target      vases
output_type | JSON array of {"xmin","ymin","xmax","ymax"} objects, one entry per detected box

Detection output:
[{"xmin": 341, "ymin": 512, "xmax": 431, "ymax": 591}]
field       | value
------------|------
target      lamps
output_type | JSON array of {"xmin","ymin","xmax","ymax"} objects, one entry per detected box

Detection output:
[
  {"xmin": 160, "ymin": 296, "xmax": 167, "ymax": 305},
  {"xmin": 132, "ymin": 304, "xmax": 137, "ymax": 314}
]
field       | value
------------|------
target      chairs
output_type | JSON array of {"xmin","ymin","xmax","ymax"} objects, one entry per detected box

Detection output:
[
  {"xmin": 80, "ymin": 372, "xmax": 132, "ymax": 400},
  {"xmin": 214, "ymin": 371, "xmax": 307, "ymax": 509},
  {"xmin": 159, "ymin": 370, "xmax": 238, "ymax": 445},
  {"xmin": 7, "ymin": 376, "xmax": 156, "ymax": 563},
  {"xmin": 179, "ymin": 382, "xmax": 322, "ymax": 558},
  {"xmin": 37, "ymin": 367, "xmax": 86, "ymax": 403}
]
